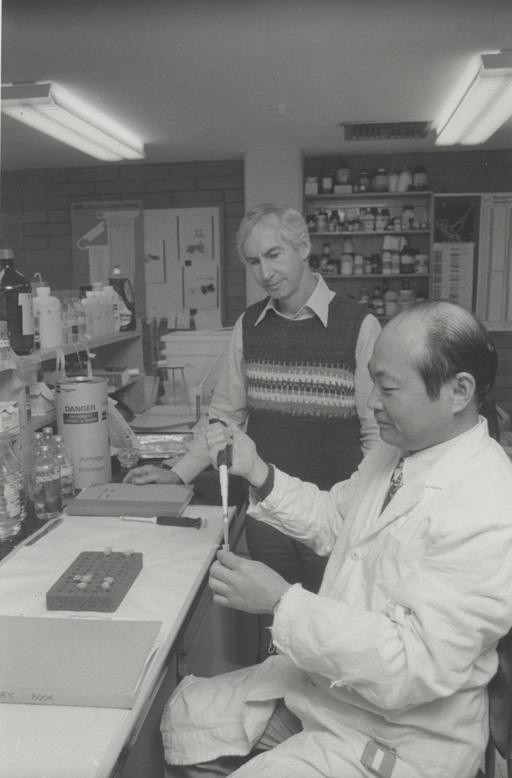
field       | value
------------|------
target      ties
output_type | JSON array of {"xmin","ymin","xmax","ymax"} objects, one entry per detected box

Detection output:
[{"xmin": 377, "ymin": 458, "xmax": 404, "ymax": 516}]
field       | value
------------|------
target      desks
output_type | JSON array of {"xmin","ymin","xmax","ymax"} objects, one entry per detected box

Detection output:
[{"xmin": 0, "ymin": 504, "xmax": 263, "ymax": 772}]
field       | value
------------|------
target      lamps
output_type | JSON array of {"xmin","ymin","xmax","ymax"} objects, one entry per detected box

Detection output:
[
  {"xmin": 4, "ymin": 82, "xmax": 145, "ymax": 162},
  {"xmin": 437, "ymin": 49, "xmax": 512, "ymax": 149}
]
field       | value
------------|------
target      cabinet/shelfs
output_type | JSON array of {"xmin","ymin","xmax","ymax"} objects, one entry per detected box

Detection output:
[
  {"xmin": 305, "ymin": 192, "xmax": 433, "ymax": 329},
  {"xmin": 143, "ymin": 207, "xmax": 228, "ymax": 333},
  {"xmin": 0, "ymin": 318, "xmax": 148, "ymax": 503}
]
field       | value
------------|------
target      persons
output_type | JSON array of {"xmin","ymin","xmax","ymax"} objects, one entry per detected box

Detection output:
[
  {"xmin": 122, "ymin": 200, "xmax": 409, "ymax": 658},
  {"xmin": 155, "ymin": 296, "xmax": 511, "ymax": 776}
]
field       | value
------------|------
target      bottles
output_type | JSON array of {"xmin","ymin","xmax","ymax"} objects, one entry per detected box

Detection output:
[
  {"xmin": 0, "ymin": 426, "xmax": 77, "ymax": 542},
  {"xmin": 0, "ymin": 248, "xmax": 36, "ymax": 356},
  {"xmin": 304, "ymin": 164, "xmax": 430, "ymax": 317},
  {"xmin": 63, "ymin": 282, "xmax": 121, "ymax": 350},
  {"xmin": 33, "ymin": 286, "xmax": 65, "ymax": 349}
]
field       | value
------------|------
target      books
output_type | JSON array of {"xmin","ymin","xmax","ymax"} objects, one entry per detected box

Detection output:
[{"xmin": 64, "ymin": 479, "xmax": 195, "ymax": 518}]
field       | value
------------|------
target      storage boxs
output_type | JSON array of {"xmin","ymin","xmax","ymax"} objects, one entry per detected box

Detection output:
[{"xmin": 158, "ymin": 326, "xmax": 231, "ymax": 398}]
